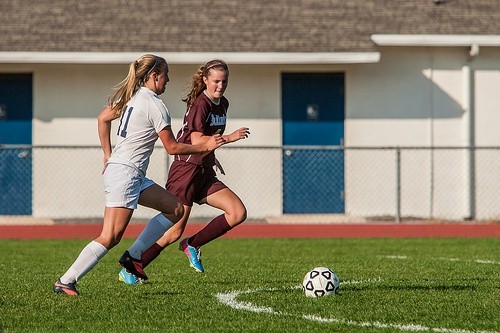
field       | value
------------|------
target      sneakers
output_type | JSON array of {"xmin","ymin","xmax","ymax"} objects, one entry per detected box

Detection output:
[
  {"xmin": 178, "ymin": 237, "xmax": 206, "ymax": 274},
  {"xmin": 118, "ymin": 250, "xmax": 149, "ymax": 279},
  {"xmin": 116, "ymin": 268, "xmax": 145, "ymax": 285},
  {"xmin": 53, "ymin": 278, "xmax": 79, "ymax": 297}
]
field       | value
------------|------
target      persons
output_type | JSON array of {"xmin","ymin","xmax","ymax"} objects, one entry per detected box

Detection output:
[
  {"xmin": 118, "ymin": 60, "xmax": 250, "ymax": 284},
  {"xmin": 53, "ymin": 54, "xmax": 226, "ymax": 295}
]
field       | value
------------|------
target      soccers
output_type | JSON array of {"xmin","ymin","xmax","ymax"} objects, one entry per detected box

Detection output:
[{"xmin": 301, "ymin": 266, "xmax": 340, "ymax": 298}]
